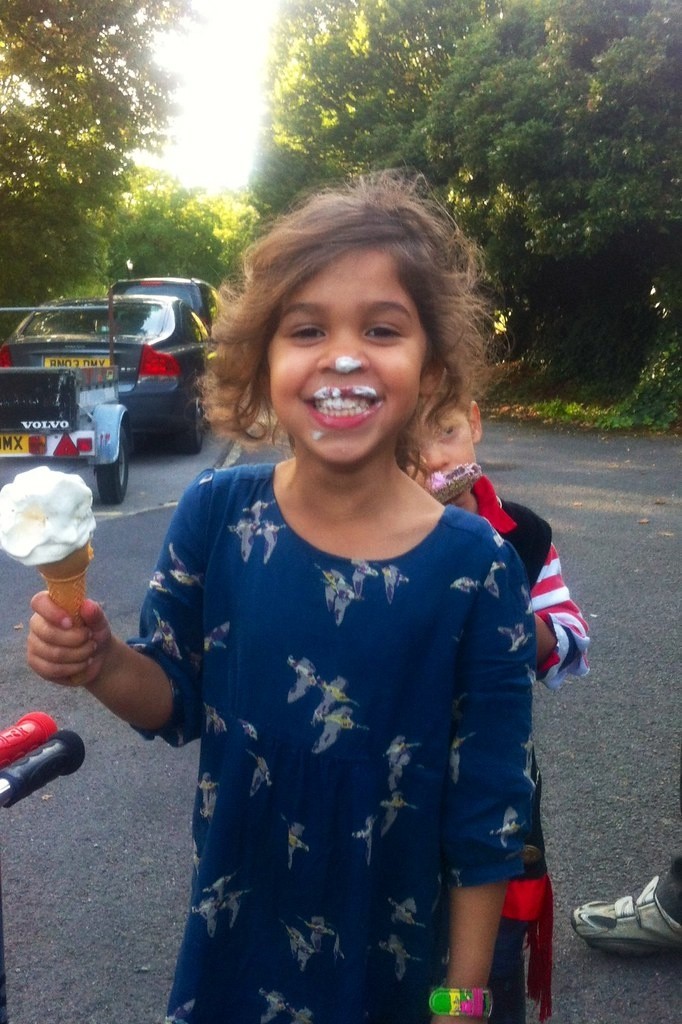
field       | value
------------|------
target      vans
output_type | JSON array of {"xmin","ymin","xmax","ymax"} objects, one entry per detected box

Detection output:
[{"xmin": 104, "ymin": 275, "xmax": 227, "ymax": 344}]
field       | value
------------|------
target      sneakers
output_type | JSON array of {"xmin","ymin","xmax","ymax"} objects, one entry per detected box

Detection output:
[{"xmin": 570, "ymin": 876, "xmax": 682, "ymax": 958}]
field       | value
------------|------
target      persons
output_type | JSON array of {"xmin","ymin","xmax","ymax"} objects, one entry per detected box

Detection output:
[
  {"xmin": 570, "ymin": 852, "xmax": 681, "ymax": 959},
  {"xmin": 24, "ymin": 169, "xmax": 539, "ymax": 1024},
  {"xmin": 406, "ymin": 395, "xmax": 592, "ymax": 1024}
]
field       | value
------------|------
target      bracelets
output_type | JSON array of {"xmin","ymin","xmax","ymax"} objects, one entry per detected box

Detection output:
[{"xmin": 429, "ymin": 987, "xmax": 495, "ymax": 1018}]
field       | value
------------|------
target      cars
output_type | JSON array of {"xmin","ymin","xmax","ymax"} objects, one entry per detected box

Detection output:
[{"xmin": 0, "ymin": 293, "xmax": 216, "ymax": 457}]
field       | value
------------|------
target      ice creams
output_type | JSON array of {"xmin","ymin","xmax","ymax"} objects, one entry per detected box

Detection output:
[
  {"xmin": 0, "ymin": 465, "xmax": 97, "ymax": 630},
  {"xmin": 425, "ymin": 463, "xmax": 482, "ymax": 503}
]
